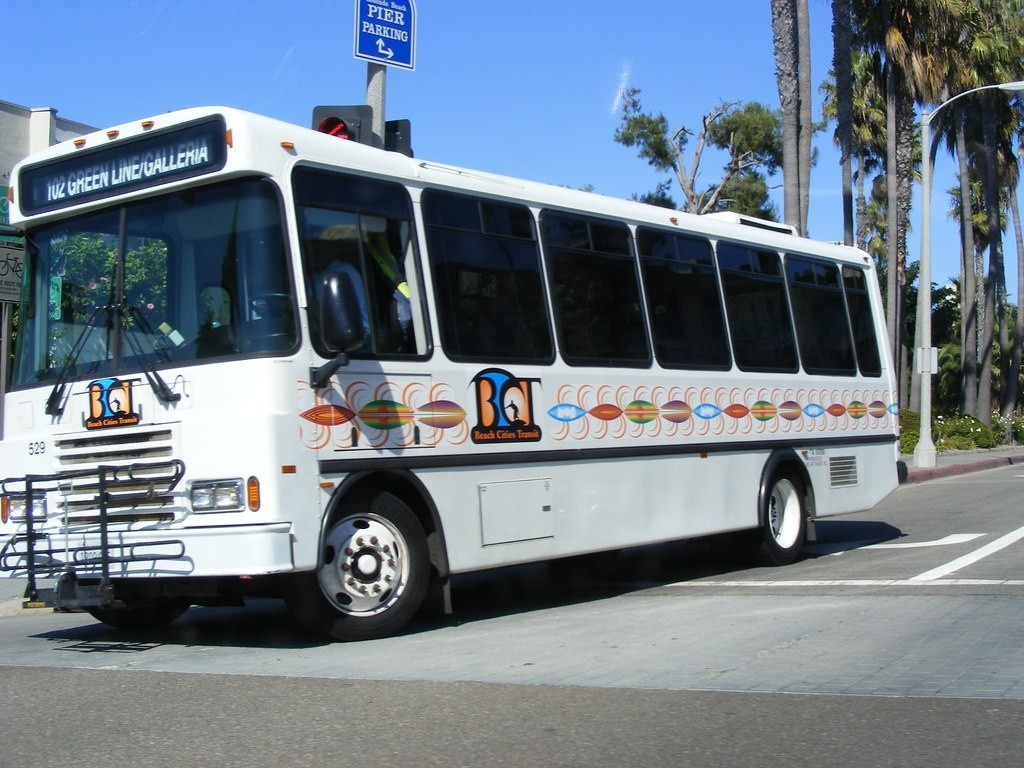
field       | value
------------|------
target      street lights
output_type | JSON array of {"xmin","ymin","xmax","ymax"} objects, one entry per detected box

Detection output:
[{"xmin": 911, "ymin": 79, "xmax": 1024, "ymax": 466}]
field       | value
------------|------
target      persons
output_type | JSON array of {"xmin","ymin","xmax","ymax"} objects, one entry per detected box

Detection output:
[{"xmin": 278, "ymin": 235, "xmax": 369, "ymax": 339}]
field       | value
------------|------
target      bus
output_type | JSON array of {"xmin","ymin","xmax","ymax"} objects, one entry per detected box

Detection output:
[{"xmin": 0, "ymin": 105, "xmax": 911, "ymax": 641}]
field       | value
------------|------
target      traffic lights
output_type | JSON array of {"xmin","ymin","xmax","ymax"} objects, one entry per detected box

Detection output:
[{"xmin": 311, "ymin": 105, "xmax": 372, "ymax": 147}]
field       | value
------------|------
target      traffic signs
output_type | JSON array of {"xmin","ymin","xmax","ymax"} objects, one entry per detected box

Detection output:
[{"xmin": 352, "ymin": 0, "xmax": 418, "ymax": 72}]
